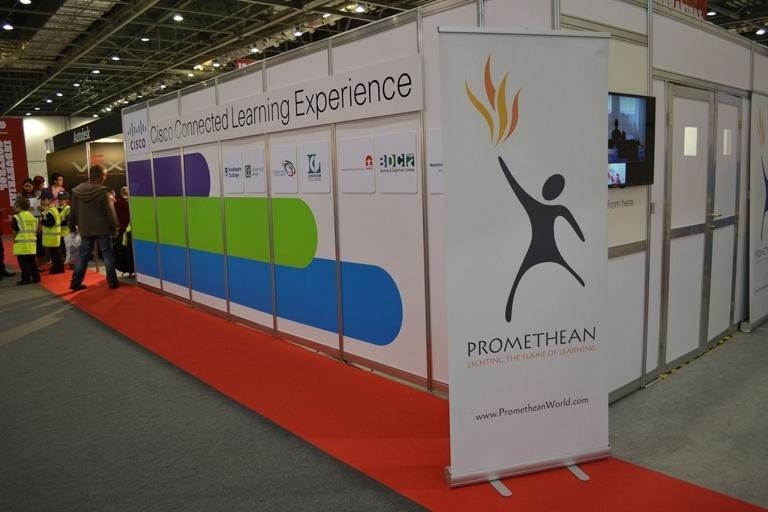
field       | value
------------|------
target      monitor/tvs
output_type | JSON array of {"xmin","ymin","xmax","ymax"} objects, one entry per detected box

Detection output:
[{"xmin": 607, "ymin": 92, "xmax": 656, "ymax": 188}]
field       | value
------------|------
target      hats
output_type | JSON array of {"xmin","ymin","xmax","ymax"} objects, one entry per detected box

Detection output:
[
  {"xmin": 56, "ymin": 191, "xmax": 70, "ymax": 199},
  {"xmin": 38, "ymin": 191, "xmax": 52, "ymax": 200},
  {"xmin": 33, "ymin": 176, "xmax": 45, "ymax": 182}
]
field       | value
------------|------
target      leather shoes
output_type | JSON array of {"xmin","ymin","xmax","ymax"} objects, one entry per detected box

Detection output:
[{"xmin": 3, "ymin": 271, "xmax": 16, "ymax": 277}]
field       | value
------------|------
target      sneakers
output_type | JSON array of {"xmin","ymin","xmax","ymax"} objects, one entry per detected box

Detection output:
[
  {"xmin": 110, "ymin": 280, "xmax": 118, "ymax": 287},
  {"xmin": 70, "ymin": 284, "xmax": 87, "ymax": 290},
  {"xmin": 17, "ymin": 281, "xmax": 31, "ymax": 285}
]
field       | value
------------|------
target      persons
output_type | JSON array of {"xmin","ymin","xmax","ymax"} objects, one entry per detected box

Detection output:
[
  {"xmin": 0, "ymin": 164, "xmax": 135, "ymax": 291},
  {"xmin": 608, "ymin": 118, "xmax": 633, "ymax": 162}
]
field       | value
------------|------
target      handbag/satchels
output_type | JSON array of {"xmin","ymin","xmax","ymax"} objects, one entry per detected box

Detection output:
[{"xmin": 63, "ymin": 232, "xmax": 82, "ymax": 265}]
